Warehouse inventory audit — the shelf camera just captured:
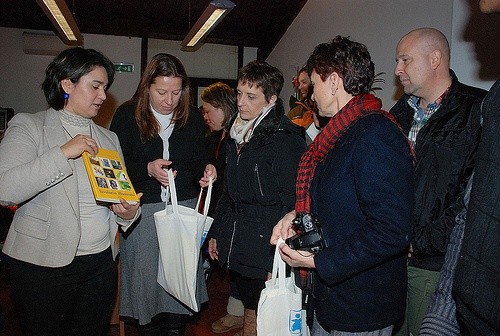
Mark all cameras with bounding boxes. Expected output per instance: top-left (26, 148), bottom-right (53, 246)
top-left (285, 212), bottom-right (328, 255)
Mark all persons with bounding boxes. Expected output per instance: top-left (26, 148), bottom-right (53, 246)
top-left (0, 0), bottom-right (500, 336)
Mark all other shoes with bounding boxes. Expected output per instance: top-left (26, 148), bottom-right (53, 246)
top-left (242, 314), bottom-right (257, 336)
top-left (212, 314), bottom-right (244, 333)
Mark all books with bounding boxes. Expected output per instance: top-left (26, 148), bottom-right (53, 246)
top-left (82, 148), bottom-right (140, 206)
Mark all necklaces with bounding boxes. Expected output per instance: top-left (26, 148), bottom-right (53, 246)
top-left (62, 122), bottom-right (93, 140)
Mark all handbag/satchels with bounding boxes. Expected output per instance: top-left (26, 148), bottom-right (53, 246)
top-left (257, 239), bottom-right (310, 336)
top-left (153, 169), bottom-right (214, 312)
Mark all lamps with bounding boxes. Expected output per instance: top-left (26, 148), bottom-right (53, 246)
top-left (180, 0), bottom-right (237, 53)
top-left (36, 0), bottom-right (84, 48)
